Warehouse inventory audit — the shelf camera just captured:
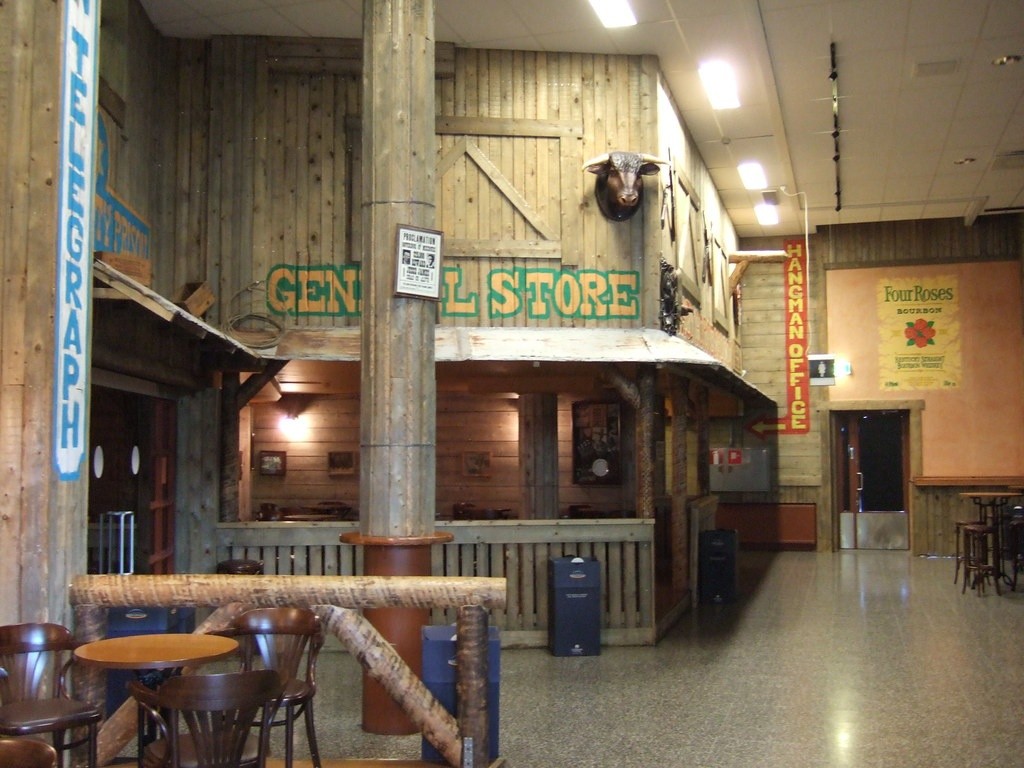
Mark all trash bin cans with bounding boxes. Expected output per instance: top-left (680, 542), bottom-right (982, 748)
top-left (420, 623), bottom-right (501, 762)
top-left (547, 555), bottom-right (602, 655)
top-left (106, 603), bottom-right (196, 721)
top-left (700, 528), bottom-right (739, 604)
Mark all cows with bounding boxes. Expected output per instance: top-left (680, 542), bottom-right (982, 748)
top-left (580, 150), bottom-right (672, 223)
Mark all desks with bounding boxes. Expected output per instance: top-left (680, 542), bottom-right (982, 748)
top-left (250, 500), bottom-right (638, 524)
top-left (959, 492), bottom-right (1024, 585)
top-left (72, 633), bottom-right (239, 768)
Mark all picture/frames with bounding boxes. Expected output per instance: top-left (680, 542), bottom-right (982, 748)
top-left (462, 450), bottom-right (492, 479)
top-left (259, 450), bottom-right (286, 476)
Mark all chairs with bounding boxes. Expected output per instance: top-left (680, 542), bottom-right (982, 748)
top-left (0, 607), bottom-right (323, 768)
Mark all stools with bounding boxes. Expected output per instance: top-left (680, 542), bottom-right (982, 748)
top-left (216, 560), bottom-right (263, 576)
top-left (954, 512), bottom-right (1024, 597)
